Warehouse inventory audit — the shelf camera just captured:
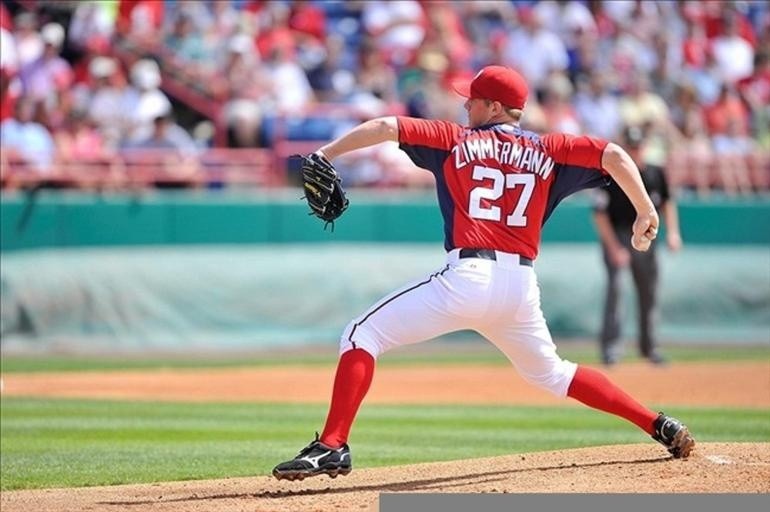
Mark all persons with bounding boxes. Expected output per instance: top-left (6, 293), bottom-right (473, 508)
top-left (269, 66), bottom-right (697, 483)
top-left (586, 123), bottom-right (685, 368)
top-left (1, 1), bottom-right (769, 200)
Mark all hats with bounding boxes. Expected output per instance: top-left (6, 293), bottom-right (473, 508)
top-left (621, 125), bottom-right (645, 147)
top-left (450, 65), bottom-right (527, 111)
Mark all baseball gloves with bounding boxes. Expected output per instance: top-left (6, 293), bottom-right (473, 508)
top-left (301, 151), bottom-right (350, 222)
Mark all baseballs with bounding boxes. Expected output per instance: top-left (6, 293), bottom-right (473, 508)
top-left (632, 235), bottom-right (651, 253)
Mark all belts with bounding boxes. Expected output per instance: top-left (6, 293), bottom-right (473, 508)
top-left (459, 247), bottom-right (532, 267)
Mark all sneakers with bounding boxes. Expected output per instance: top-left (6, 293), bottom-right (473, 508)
top-left (271, 431), bottom-right (353, 482)
top-left (600, 340), bottom-right (667, 367)
top-left (651, 409), bottom-right (695, 459)
